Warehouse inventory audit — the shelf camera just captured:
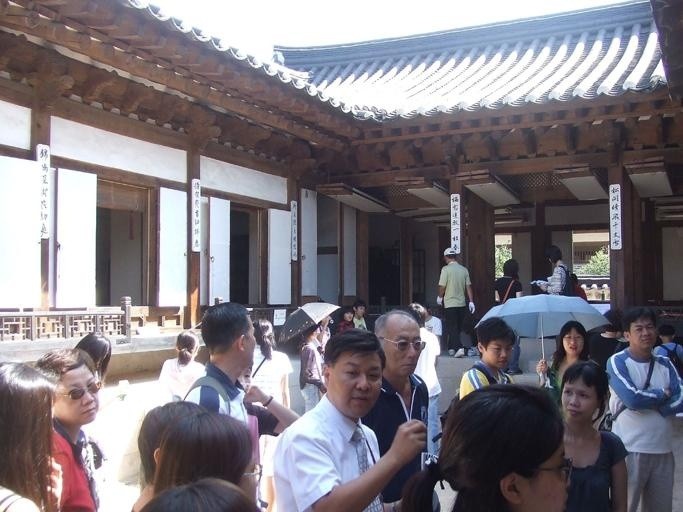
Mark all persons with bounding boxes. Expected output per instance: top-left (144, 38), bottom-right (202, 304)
top-left (435, 248), bottom-right (476, 358)
top-left (492, 259), bottom-right (524, 374)
top-left (1, 332), bottom-right (111, 511)
top-left (532, 245), bottom-right (569, 296)
top-left (132, 301), bottom-right (682, 511)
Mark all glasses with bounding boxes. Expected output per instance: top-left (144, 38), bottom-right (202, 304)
top-left (524, 456), bottom-right (572, 482)
top-left (62, 377), bottom-right (101, 399)
top-left (241, 463), bottom-right (262, 484)
top-left (378, 335), bottom-right (425, 351)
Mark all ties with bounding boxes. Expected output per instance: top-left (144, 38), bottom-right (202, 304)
top-left (352, 425), bottom-right (384, 512)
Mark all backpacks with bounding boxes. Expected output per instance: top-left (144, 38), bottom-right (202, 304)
top-left (658, 343), bottom-right (679, 372)
top-left (557, 265), bottom-right (587, 301)
top-left (432, 365), bottom-right (497, 443)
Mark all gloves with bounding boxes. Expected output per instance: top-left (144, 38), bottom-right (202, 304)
top-left (436, 295), bottom-right (442, 305)
top-left (468, 301), bottom-right (475, 314)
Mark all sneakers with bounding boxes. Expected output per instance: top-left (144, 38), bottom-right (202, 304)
top-left (453, 348), bottom-right (464, 357)
top-left (448, 348), bottom-right (455, 355)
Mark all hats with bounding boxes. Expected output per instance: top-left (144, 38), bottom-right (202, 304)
top-left (443, 247), bottom-right (455, 256)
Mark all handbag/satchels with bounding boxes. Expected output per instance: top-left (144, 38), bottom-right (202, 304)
top-left (596, 409), bottom-right (612, 432)
